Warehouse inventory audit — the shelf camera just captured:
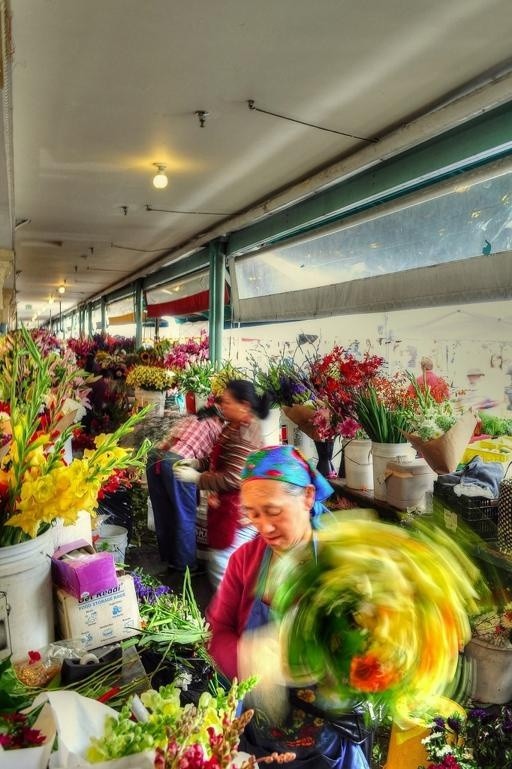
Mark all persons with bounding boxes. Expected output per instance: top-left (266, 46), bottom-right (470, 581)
top-left (203, 445), bottom-right (368, 769)
top-left (458, 367), bottom-right (485, 402)
top-left (171, 379), bottom-right (263, 593)
top-left (485, 353), bottom-right (506, 417)
top-left (146, 405), bottom-right (225, 578)
top-left (404, 356), bottom-right (450, 402)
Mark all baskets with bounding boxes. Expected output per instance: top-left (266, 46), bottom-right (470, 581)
top-left (497, 478), bottom-right (512, 555)
top-left (463, 434), bottom-right (511, 467)
top-left (432, 481), bottom-right (498, 546)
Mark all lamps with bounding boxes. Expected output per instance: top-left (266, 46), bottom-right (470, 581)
top-left (152, 163), bottom-right (168, 189)
top-left (27, 286), bottom-right (65, 326)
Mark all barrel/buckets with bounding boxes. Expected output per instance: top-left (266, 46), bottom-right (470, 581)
top-left (93, 523), bottom-right (128, 564)
top-left (341, 438), bottom-right (374, 490)
top-left (194, 489), bottom-right (216, 560)
top-left (258, 407), bottom-right (281, 446)
top-left (371, 441), bottom-right (417, 500)
top-left (194, 392), bottom-right (207, 413)
top-left (280, 408), bottom-right (314, 458)
top-left (464, 637), bottom-right (512, 705)
top-left (145, 496), bottom-right (156, 532)
top-left (134, 388), bottom-right (166, 420)
top-left (0, 524), bottom-right (54, 661)
